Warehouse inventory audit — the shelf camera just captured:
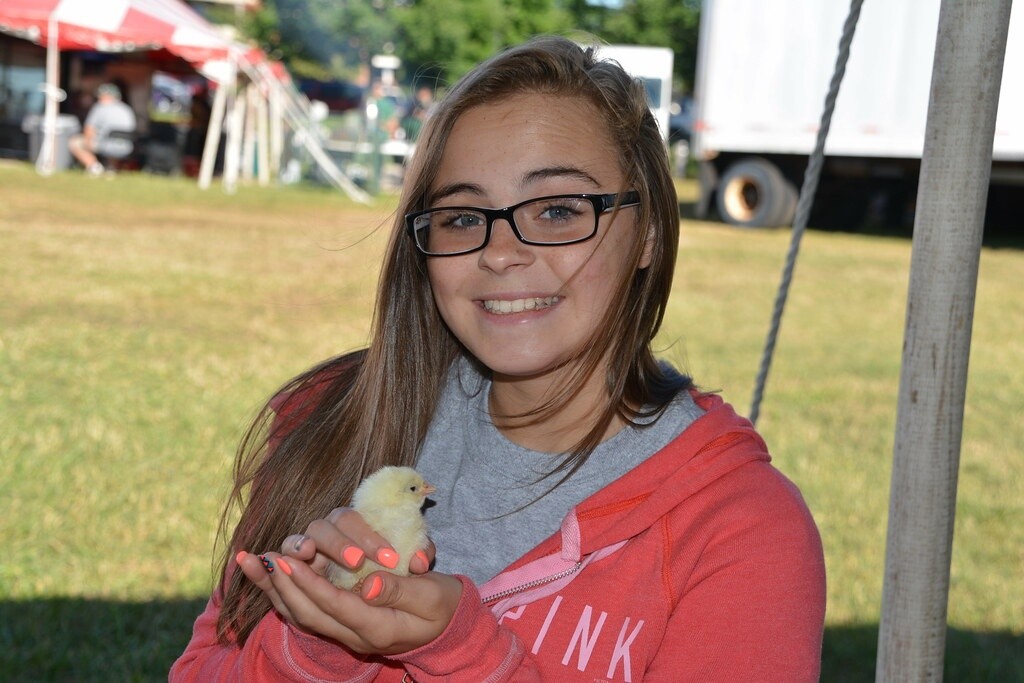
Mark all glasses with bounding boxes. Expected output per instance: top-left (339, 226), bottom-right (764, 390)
top-left (405, 190), bottom-right (641, 256)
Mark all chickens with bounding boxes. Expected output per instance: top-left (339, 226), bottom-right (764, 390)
top-left (303, 465), bottom-right (438, 594)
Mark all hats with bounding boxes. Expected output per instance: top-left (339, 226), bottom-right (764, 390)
top-left (96, 83), bottom-right (121, 97)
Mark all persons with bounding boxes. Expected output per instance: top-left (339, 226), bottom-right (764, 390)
top-left (168, 37), bottom-right (825, 683)
top-left (67, 84), bottom-right (138, 174)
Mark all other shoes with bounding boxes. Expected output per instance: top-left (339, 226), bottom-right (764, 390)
top-left (88, 163), bottom-right (105, 176)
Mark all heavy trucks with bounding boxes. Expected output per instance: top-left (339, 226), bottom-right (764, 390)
top-left (557, 0), bottom-right (1023, 227)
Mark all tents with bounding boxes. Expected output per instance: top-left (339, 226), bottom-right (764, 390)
top-left (0, 0), bottom-right (368, 200)
